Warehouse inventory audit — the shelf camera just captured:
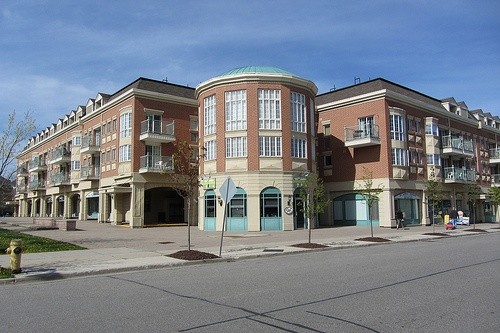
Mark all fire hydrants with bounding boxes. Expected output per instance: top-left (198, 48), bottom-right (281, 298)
top-left (4, 239), bottom-right (23, 274)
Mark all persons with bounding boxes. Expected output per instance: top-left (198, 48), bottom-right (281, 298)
top-left (449, 206), bottom-right (459, 228)
top-left (395, 208), bottom-right (405, 230)
